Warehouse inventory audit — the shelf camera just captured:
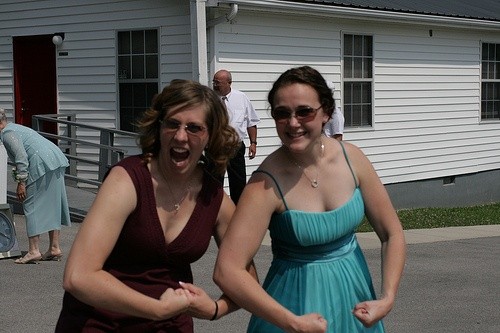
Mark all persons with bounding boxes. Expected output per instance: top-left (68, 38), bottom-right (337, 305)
top-left (55, 78), bottom-right (260, 333)
top-left (321, 80), bottom-right (345, 141)
top-left (206, 69), bottom-right (260, 207)
top-left (211, 65), bottom-right (407, 333)
top-left (0, 110), bottom-right (72, 265)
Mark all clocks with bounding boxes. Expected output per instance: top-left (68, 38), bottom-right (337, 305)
top-left (0, 204), bottom-right (22, 259)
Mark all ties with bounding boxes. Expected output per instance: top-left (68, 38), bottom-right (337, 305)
top-left (222, 97), bottom-right (227, 110)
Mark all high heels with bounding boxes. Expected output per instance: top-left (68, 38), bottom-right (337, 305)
top-left (14, 256), bottom-right (42, 264)
top-left (41, 253), bottom-right (62, 262)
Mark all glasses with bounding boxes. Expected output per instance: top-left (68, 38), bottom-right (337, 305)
top-left (271, 104), bottom-right (322, 119)
top-left (163, 119), bottom-right (206, 135)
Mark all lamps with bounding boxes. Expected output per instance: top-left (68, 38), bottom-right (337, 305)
top-left (52, 32), bottom-right (64, 45)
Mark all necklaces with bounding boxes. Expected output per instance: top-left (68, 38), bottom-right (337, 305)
top-left (282, 139), bottom-right (325, 187)
top-left (158, 159), bottom-right (194, 210)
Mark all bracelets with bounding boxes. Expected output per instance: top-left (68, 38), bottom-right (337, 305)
top-left (251, 141), bottom-right (256, 144)
top-left (210, 299), bottom-right (218, 321)
